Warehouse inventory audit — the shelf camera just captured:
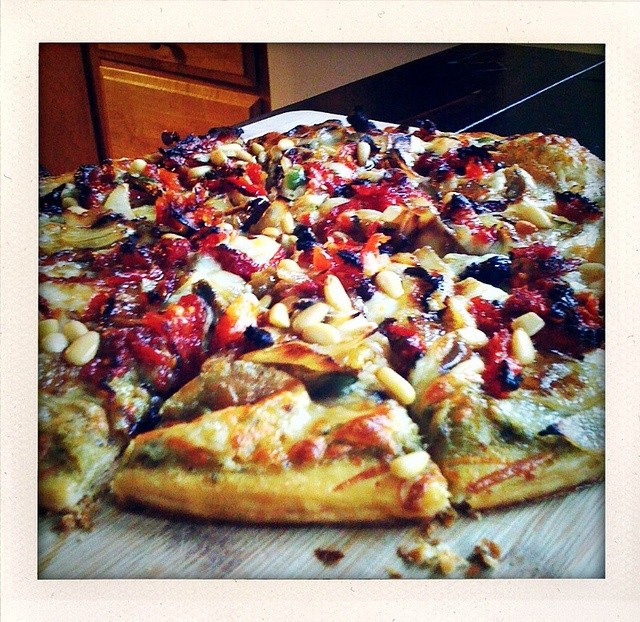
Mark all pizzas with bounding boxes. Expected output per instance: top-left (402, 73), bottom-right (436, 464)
top-left (38, 110), bottom-right (604, 527)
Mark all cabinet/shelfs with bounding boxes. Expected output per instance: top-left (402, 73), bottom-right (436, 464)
top-left (80, 38), bottom-right (264, 162)
